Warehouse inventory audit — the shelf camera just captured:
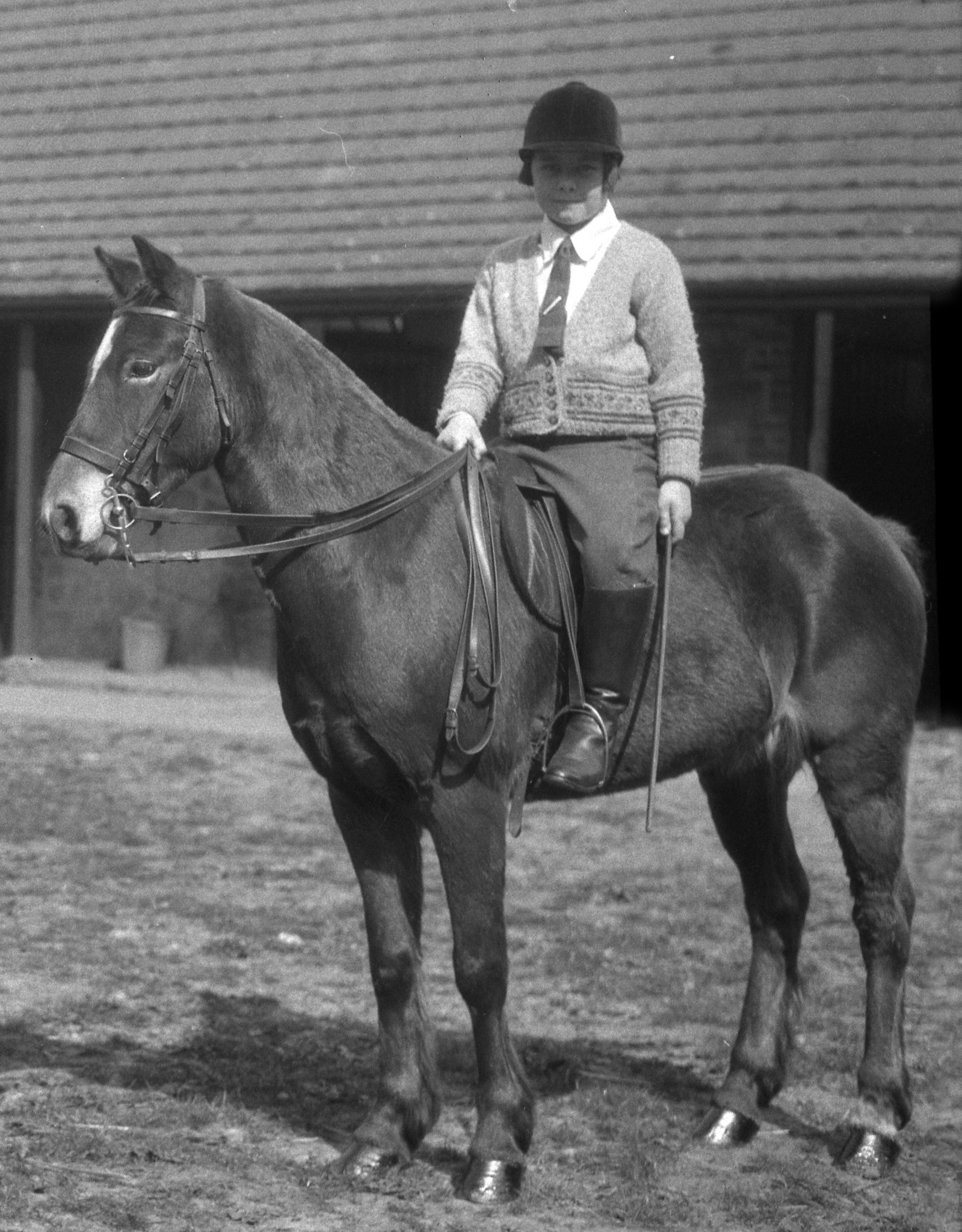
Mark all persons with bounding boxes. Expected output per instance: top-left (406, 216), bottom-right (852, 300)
top-left (433, 81), bottom-right (707, 793)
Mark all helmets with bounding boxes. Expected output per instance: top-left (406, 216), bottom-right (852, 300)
top-left (518, 82), bottom-right (623, 170)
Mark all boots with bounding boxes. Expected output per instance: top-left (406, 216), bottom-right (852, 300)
top-left (542, 585), bottom-right (654, 794)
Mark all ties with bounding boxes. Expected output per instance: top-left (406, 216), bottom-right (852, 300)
top-left (534, 236), bottom-right (571, 357)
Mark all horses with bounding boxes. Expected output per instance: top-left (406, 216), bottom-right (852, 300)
top-left (38, 234), bottom-right (928, 1208)
